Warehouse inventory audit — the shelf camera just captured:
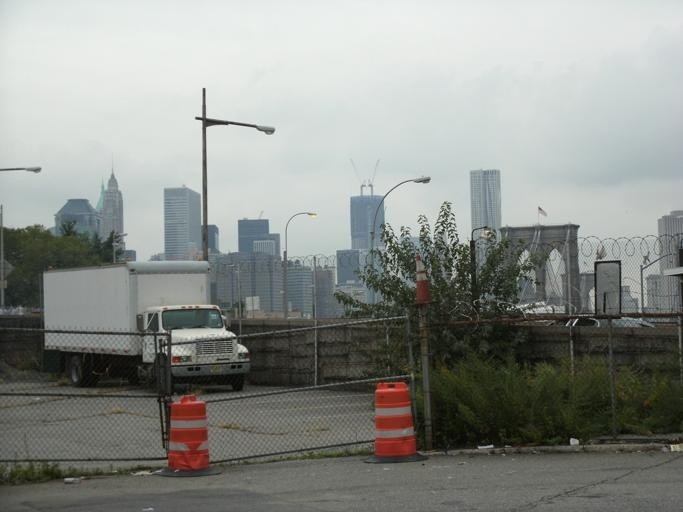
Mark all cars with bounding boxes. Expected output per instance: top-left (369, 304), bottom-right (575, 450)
top-left (545, 317), bottom-right (655, 328)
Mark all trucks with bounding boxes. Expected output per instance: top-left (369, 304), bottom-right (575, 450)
top-left (42, 261), bottom-right (250, 393)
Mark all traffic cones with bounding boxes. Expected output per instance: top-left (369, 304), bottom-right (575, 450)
top-left (410, 254), bottom-right (438, 307)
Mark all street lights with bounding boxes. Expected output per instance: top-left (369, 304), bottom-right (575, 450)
top-left (195, 115), bottom-right (275, 259)
top-left (1, 166), bottom-right (43, 174)
top-left (469, 226), bottom-right (494, 310)
top-left (282, 212), bottom-right (317, 318)
top-left (365, 177), bottom-right (431, 305)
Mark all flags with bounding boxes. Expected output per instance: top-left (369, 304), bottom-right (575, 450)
top-left (538, 207), bottom-right (548, 217)
